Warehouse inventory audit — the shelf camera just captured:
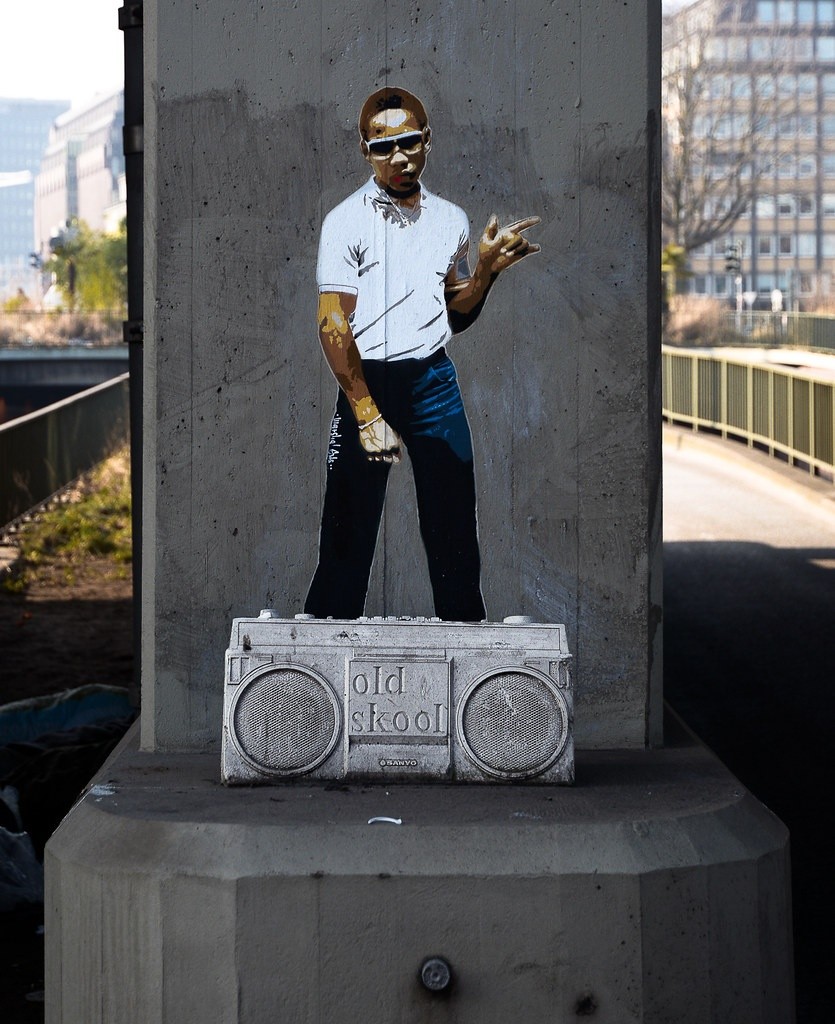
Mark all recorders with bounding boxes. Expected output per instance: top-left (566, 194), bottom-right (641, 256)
top-left (221, 607), bottom-right (575, 789)
